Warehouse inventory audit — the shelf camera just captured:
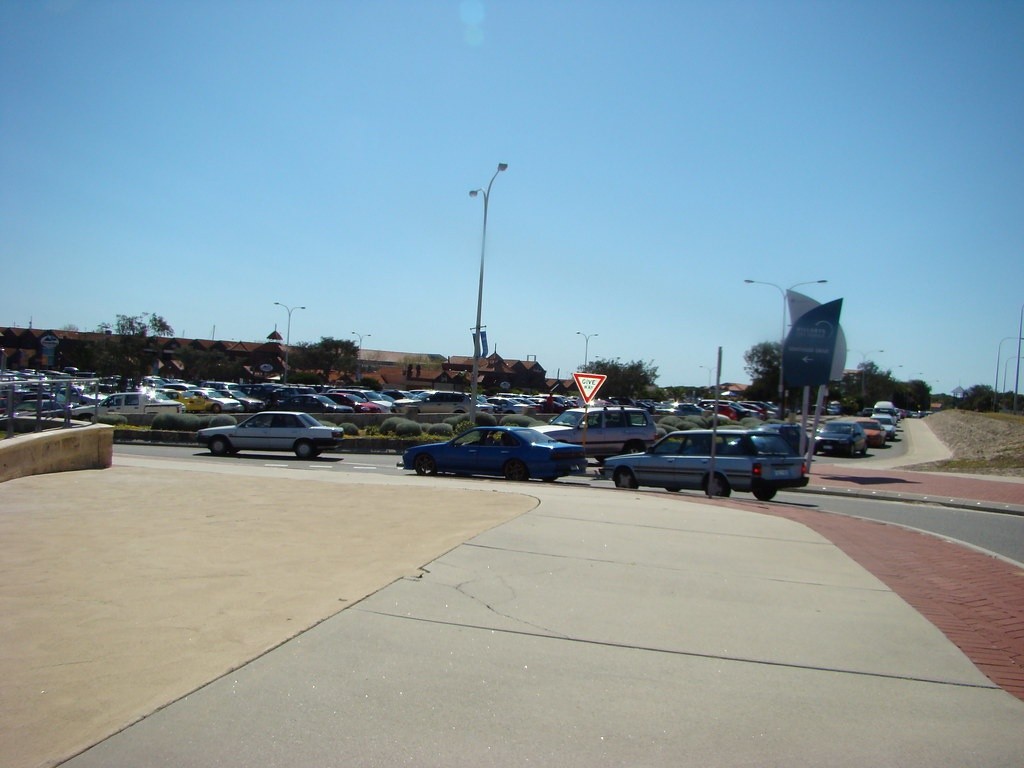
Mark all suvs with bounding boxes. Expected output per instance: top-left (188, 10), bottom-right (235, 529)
top-left (530, 406), bottom-right (657, 463)
top-left (390, 391), bottom-right (471, 414)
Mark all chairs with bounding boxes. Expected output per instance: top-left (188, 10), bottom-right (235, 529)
top-left (606, 414), bottom-right (614, 426)
top-left (500, 433), bottom-right (512, 446)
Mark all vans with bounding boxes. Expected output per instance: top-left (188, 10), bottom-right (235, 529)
top-left (873, 401), bottom-right (895, 417)
top-left (826, 400), bottom-right (841, 414)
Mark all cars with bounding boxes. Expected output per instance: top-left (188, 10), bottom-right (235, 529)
top-left (476, 392), bottom-right (790, 421)
top-left (155, 389), bottom-right (212, 413)
top-left (160, 378), bottom-right (415, 414)
top-left (812, 413), bottom-right (895, 457)
top-left (894, 407), bottom-right (926, 420)
top-left (863, 408), bottom-right (873, 416)
top-left (0, 367), bottom-right (161, 422)
top-left (196, 411), bottom-right (345, 461)
top-left (403, 425), bottom-right (587, 482)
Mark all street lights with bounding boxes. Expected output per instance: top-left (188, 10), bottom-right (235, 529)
top-left (274, 303), bottom-right (305, 385)
top-left (576, 332), bottom-right (598, 370)
top-left (469, 162), bottom-right (507, 424)
top-left (352, 332), bottom-right (371, 384)
top-left (745, 279), bottom-right (829, 420)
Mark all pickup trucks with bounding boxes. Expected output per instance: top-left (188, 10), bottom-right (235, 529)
top-left (71, 391), bottom-right (186, 417)
top-left (600, 428), bottom-right (809, 502)
